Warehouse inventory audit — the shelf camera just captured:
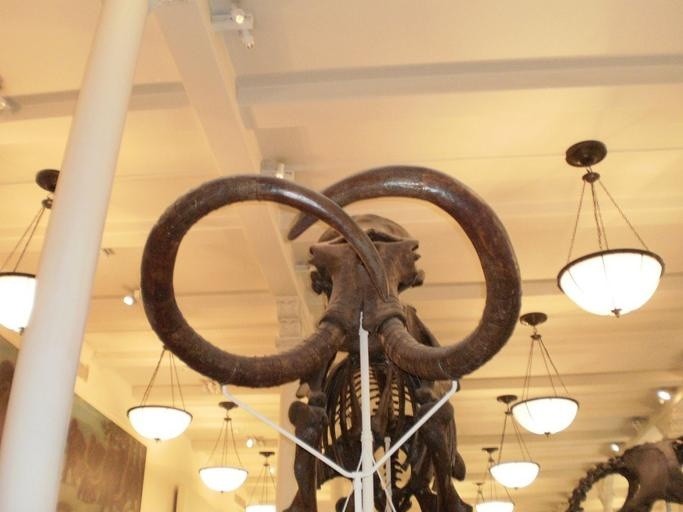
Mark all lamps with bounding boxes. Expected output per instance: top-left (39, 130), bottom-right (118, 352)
top-left (229, 4), bottom-right (256, 51)
top-left (472, 310), bottom-right (581, 511)
top-left (555, 139), bottom-right (666, 322)
top-left (125, 342), bottom-right (280, 512)
top-left (0, 169), bottom-right (59, 337)
